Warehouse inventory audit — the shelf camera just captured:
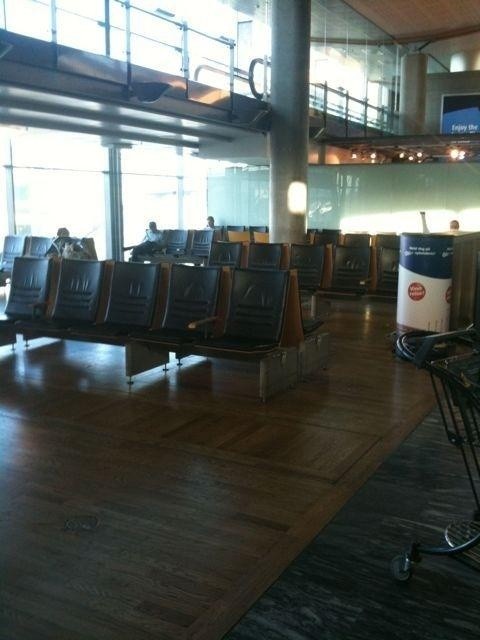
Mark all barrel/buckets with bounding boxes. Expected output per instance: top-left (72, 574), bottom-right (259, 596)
top-left (397, 232), bottom-right (453, 342)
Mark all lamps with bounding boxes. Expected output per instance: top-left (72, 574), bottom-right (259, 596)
top-left (122, 80), bottom-right (171, 103)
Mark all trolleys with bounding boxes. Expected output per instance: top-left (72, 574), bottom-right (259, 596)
top-left (389, 321), bottom-right (480, 581)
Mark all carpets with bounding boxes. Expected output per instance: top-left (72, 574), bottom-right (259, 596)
top-left (216, 384), bottom-right (480, 640)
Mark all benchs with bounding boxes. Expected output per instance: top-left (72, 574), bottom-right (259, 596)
top-left (1, 255), bottom-right (333, 398)
top-left (1, 226), bottom-right (398, 305)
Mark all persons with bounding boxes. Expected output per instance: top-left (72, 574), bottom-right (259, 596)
top-left (449, 220), bottom-right (460, 235)
top-left (131, 222), bottom-right (168, 259)
top-left (201, 217), bottom-right (220, 231)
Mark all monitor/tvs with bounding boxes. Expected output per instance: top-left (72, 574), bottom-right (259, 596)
top-left (440, 92), bottom-right (480, 134)
